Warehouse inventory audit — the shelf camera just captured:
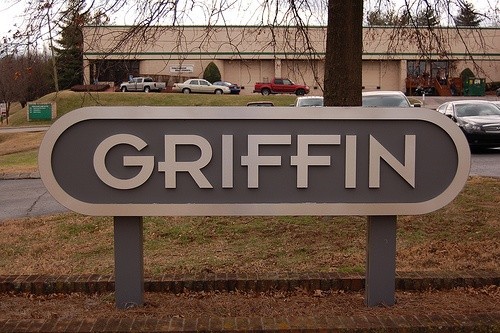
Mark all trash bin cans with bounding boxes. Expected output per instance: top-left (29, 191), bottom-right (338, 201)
top-left (462, 77), bottom-right (486, 96)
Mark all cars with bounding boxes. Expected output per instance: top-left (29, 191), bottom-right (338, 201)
top-left (171, 78), bottom-right (231, 95)
top-left (434, 99), bottom-right (500, 153)
top-left (211, 80), bottom-right (241, 95)
top-left (289, 95), bottom-right (323, 107)
top-left (245, 101), bottom-right (274, 107)
top-left (362, 90), bottom-right (422, 109)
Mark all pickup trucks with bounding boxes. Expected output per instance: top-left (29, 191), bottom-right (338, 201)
top-left (119, 76), bottom-right (167, 93)
top-left (252, 77), bottom-right (310, 96)
